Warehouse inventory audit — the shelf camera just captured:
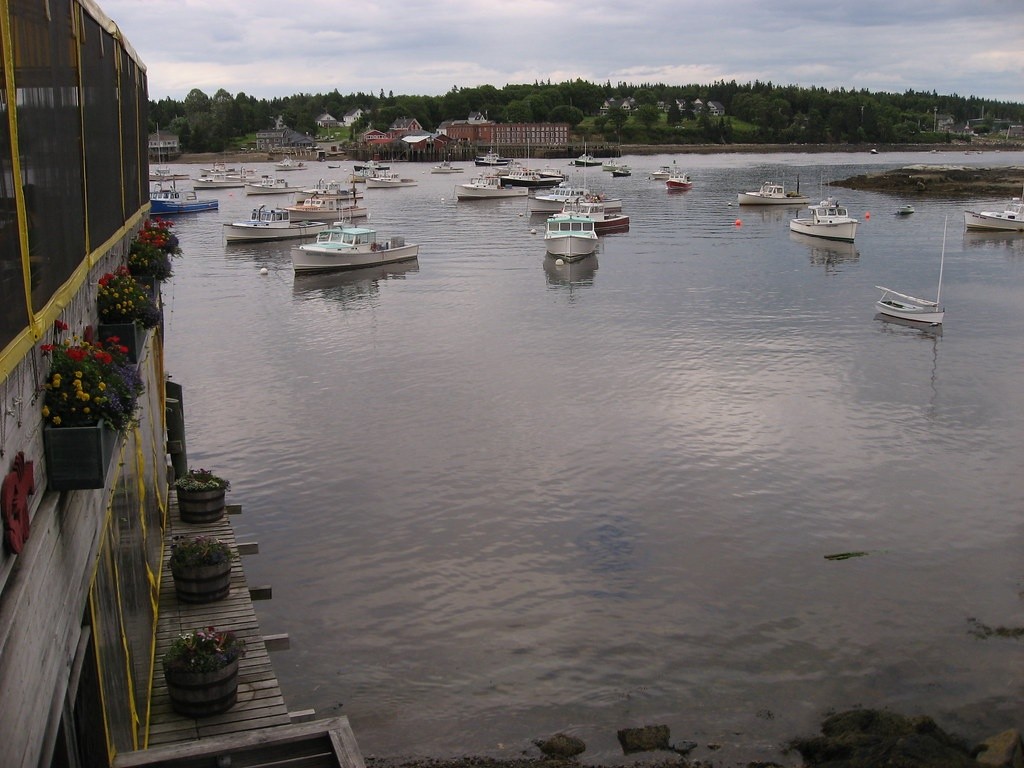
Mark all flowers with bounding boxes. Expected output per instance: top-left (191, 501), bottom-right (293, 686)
top-left (38, 317), bottom-right (147, 442)
top-left (174, 468), bottom-right (232, 493)
top-left (169, 534), bottom-right (236, 568)
top-left (161, 624), bottom-right (248, 677)
top-left (96, 213), bottom-right (183, 332)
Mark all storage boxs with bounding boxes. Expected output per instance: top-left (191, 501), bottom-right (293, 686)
top-left (129, 272), bottom-right (160, 302)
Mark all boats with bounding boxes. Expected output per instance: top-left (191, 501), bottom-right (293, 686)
top-left (455, 177), bottom-right (529, 200)
top-left (553, 202), bottom-right (629, 230)
top-left (529, 188), bottom-right (622, 213)
top-left (897, 204), bottom-right (916, 215)
top-left (737, 183), bottom-right (807, 205)
top-left (543, 214), bottom-right (598, 257)
top-left (603, 159), bottom-right (622, 171)
top-left (149, 154), bottom-right (564, 224)
top-left (224, 203), bottom-right (330, 243)
top-left (789, 199), bottom-right (859, 243)
top-left (613, 169), bottom-right (631, 177)
top-left (575, 154), bottom-right (602, 166)
top-left (666, 171), bottom-right (691, 190)
top-left (290, 226), bottom-right (419, 273)
top-left (874, 284), bottom-right (947, 326)
top-left (652, 160), bottom-right (691, 179)
top-left (961, 200), bottom-right (1024, 232)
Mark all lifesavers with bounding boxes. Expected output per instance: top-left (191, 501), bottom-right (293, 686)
top-left (175, 193), bottom-right (179, 198)
top-left (532, 176), bottom-right (535, 180)
top-left (160, 190), bottom-right (171, 192)
top-left (586, 194), bottom-right (590, 199)
top-left (371, 242), bottom-right (377, 251)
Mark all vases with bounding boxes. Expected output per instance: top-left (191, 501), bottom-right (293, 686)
top-left (42, 415), bottom-right (114, 489)
top-left (164, 655), bottom-right (240, 717)
top-left (172, 559), bottom-right (230, 603)
top-left (176, 486), bottom-right (226, 522)
top-left (98, 318), bottom-right (146, 363)
